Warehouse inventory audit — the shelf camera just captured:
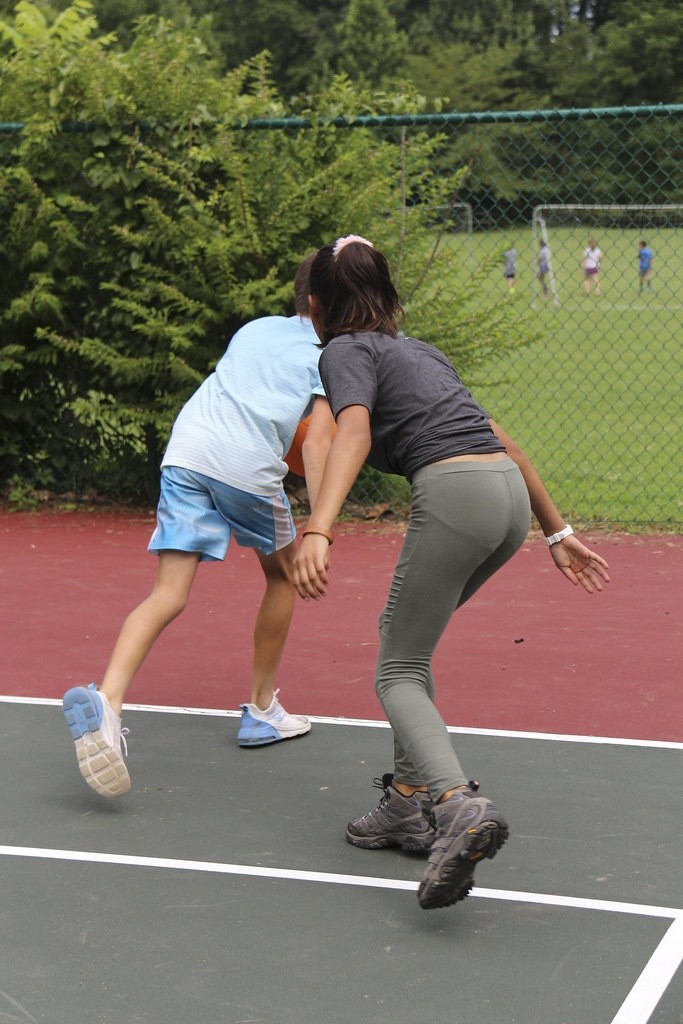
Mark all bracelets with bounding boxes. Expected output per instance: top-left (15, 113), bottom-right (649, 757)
top-left (301, 526), bottom-right (334, 546)
top-left (544, 523), bottom-right (575, 546)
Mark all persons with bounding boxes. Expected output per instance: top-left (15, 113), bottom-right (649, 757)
top-left (61, 255), bottom-right (334, 796)
top-left (501, 242), bottom-right (518, 292)
top-left (581, 240), bottom-right (604, 297)
top-left (535, 240), bottom-right (553, 297)
top-left (637, 239), bottom-right (654, 295)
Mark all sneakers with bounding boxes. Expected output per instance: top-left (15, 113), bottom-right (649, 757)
top-left (237, 693), bottom-right (311, 747)
top-left (346, 773), bottom-right (438, 852)
top-left (417, 786), bottom-right (509, 910)
top-left (61, 686), bottom-right (131, 795)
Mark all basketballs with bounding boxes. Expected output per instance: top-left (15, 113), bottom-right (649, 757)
top-left (281, 411), bottom-right (339, 479)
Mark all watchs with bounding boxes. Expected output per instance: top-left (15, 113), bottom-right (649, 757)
top-left (288, 234), bottom-right (613, 911)
